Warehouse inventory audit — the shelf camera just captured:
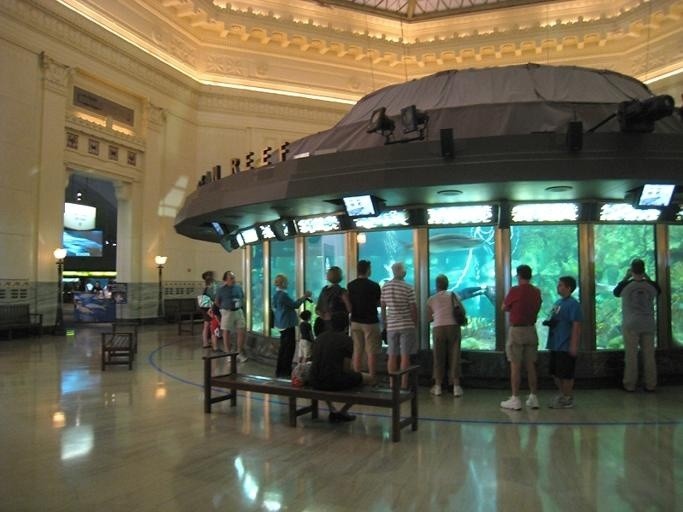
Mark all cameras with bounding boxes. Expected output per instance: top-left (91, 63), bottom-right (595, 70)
top-left (543, 316), bottom-right (559, 328)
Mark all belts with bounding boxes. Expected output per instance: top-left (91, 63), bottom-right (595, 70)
top-left (223, 308), bottom-right (242, 312)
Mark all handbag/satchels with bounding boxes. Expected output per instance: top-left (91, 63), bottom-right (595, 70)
top-left (197, 295), bottom-right (216, 309)
top-left (314, 317), bottom-right (324, 336)
top-left (453, 306), bottom-right (468, 326)
top-left (328, 296), bottom-right (346, 312)
top-left (291, 361), bottom-right (313, 388)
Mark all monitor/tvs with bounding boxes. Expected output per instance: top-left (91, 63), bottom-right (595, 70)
top-left (60, 231), bottom-right (104, 257)
top-left (638, 184), bottom-right (676, 210)
top-left (211, 222), bottom-right (227, 236)
top-left (342, 195), bottom-right (374, 218)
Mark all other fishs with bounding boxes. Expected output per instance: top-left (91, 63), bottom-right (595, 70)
top-left (393, 234), bottom-right (538, 306)
top-left (481, 326), bottom-right (495, 337)
top-left (538, 267), bottom-right (561, 276)
top-left (596, 282), bottom-right (617, 294)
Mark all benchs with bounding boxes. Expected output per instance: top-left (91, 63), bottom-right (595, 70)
top-left (0, 302), bottom-right (43, 340)
top-left (163, 298), bottom-right (202, 323)
top-left (100, 323), bottom-right (138, 370)
top-left (201, 351), bottom-right (420, 443)
top-left (176, 311), bottom-right (203, 336)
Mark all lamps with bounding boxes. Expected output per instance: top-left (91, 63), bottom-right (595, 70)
top-left (366, 103), bottom-right (430, 145)
top-left (618, 90), bottom-right (676, 135)
top-left (563, 117), bottom-right (584, 151)
top-left (153, 255), bottom-right (168, 325)
top-left (51, 247), bottom-right (67, 336)
top-left (438, 125), bottom-right (454, 159)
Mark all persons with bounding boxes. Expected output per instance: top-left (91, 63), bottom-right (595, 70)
top-left (613, 258), bottom-right (662, 391)
top-left (543, 277), bottom-right (586, 408)
top-left (271, 260), bottom-right (417, 423)
top-left (500, 265), bottom-right (542, 409)
top-left (425, 273), bottom-right (465, 397)
top-left (92, 281), bottom-right (104, 296)
top-left (197, 270), bottom-right (249, 363)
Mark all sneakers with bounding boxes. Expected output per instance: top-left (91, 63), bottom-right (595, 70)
top-left (237, 354), bottom-right (250, 363)
top-left (500, 400), bottom-right (521, 411)
top-left (400, 387), bottom-right (411, 394)
top-left (526, 398), bottom-right (539, 409)
top-left (454, 385), bottom-right (464, 396)
top-left (329, 411), bottom-right (356, 422)
top-left (550, 393), bottom-right (576, 408)
top-left (430, 384), bottom-right (442, 396)
